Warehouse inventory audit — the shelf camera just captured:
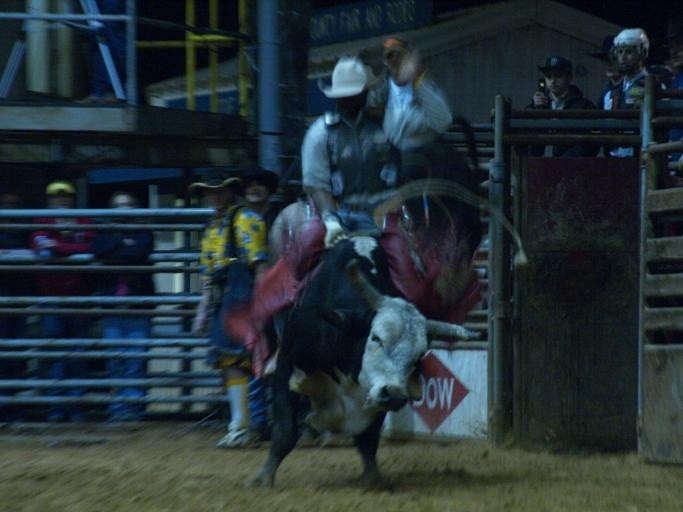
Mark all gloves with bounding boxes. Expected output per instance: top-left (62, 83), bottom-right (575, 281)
top-left (323, 215), bottom-right (348, 248)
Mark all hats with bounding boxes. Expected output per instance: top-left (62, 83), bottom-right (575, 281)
top-left (239, 165), bottom-right (279, 192)
top-left (381, 37), bottom-right (411, 59)
top-left (185, 166), bottom-right (241, 201)
top-left (536, 56), bottom-right (573, 76)
top-left (315, 56), bottom-right (369, 102)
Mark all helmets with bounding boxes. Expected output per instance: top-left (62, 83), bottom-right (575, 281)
top-left (611, 26), bottom-right (651, 58)
top-left (44, 179), bottom-right (77, 198)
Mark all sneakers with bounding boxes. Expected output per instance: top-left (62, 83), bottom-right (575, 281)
top-left (215, 424), bottom-right (253, 450)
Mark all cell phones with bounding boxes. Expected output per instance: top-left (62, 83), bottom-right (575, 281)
top-left (539, 77), bottom-right (545, 94)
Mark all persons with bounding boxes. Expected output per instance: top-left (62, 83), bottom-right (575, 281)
top-left (0, 190), bottom-right (38, 422)
top-left (88, 192), bottom-right (156, 422)
top-left (27, 181), bottom-right (98, 423)
top-left (289, 57), bottom-right (455, 388)
top-left (233, 167), bottom-right (309, 449)
top-left (368, 36), bottom-right (437, 212)
top-left (513, 26), bottom-right (683, 177)
top-left (186, 164), bottom-right (272, 448)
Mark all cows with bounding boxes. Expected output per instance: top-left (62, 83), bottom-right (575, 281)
top-left (243, 234), bottom-right (484, 490)
